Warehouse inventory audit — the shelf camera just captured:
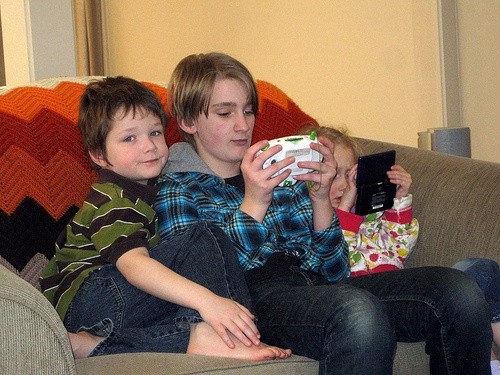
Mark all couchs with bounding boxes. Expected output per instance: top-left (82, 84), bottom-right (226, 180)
top-left (0, 77), bottom-right (500, 375)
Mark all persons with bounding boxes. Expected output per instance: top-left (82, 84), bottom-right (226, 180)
top-left (36, 74), bottom-right (292, 361)
top-left (296, 125), bottom-right (500, 375)
top-left (155, 51), bottom-right (493, 375)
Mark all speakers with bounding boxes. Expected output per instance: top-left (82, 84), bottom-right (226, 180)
top-left (417, 126), bottom-right (471, 158)
